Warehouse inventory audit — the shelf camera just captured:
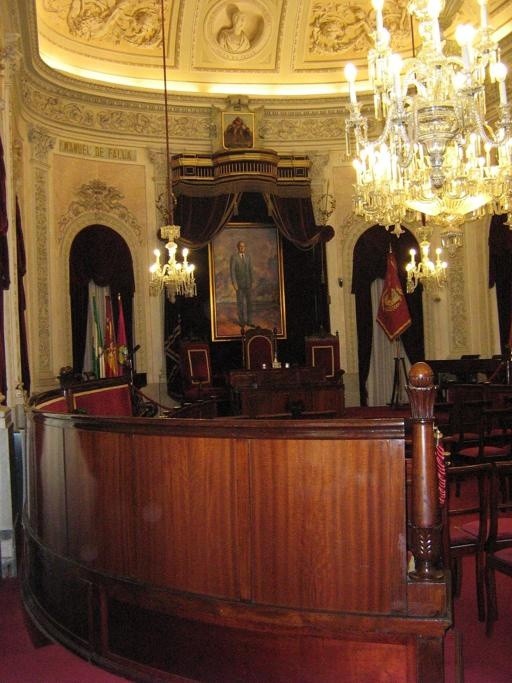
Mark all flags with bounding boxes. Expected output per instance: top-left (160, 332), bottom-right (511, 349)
top-left (91, 292), bottom-right (103, 376)
top-left (102, 296), bottom-right (119, 376)
top-left (115, 298), bottom-right (128, 375)
top-left (373, 250), bottom-right (411, 345)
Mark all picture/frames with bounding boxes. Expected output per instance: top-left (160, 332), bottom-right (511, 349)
top-left (207, 223), bottom-right (287, 343)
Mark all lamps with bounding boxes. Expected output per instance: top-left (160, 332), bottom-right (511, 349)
top-left (144, 2), bottom-right (199, 300)
top-left (342, 1), bottom-right (512, 298)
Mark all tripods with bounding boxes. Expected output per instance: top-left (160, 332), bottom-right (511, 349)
top-left (391, 336), bottom-right (409, 407)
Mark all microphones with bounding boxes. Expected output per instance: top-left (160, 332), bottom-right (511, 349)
top-left (127, 345), bottom-right (141, 359)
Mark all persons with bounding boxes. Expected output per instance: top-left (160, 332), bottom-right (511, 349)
top-left (230, 238), bottom-right (255, 326)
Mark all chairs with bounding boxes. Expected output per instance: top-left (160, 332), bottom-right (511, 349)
top-left (178, 324), bottom-right (346, 389)
top-left (424, 399), bottom-right (512, 638)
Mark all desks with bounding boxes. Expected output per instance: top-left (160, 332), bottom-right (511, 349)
top-left (444, 382), bottom-right (511, 403)
top-left (224, 367), bottom-right (327, 411)
